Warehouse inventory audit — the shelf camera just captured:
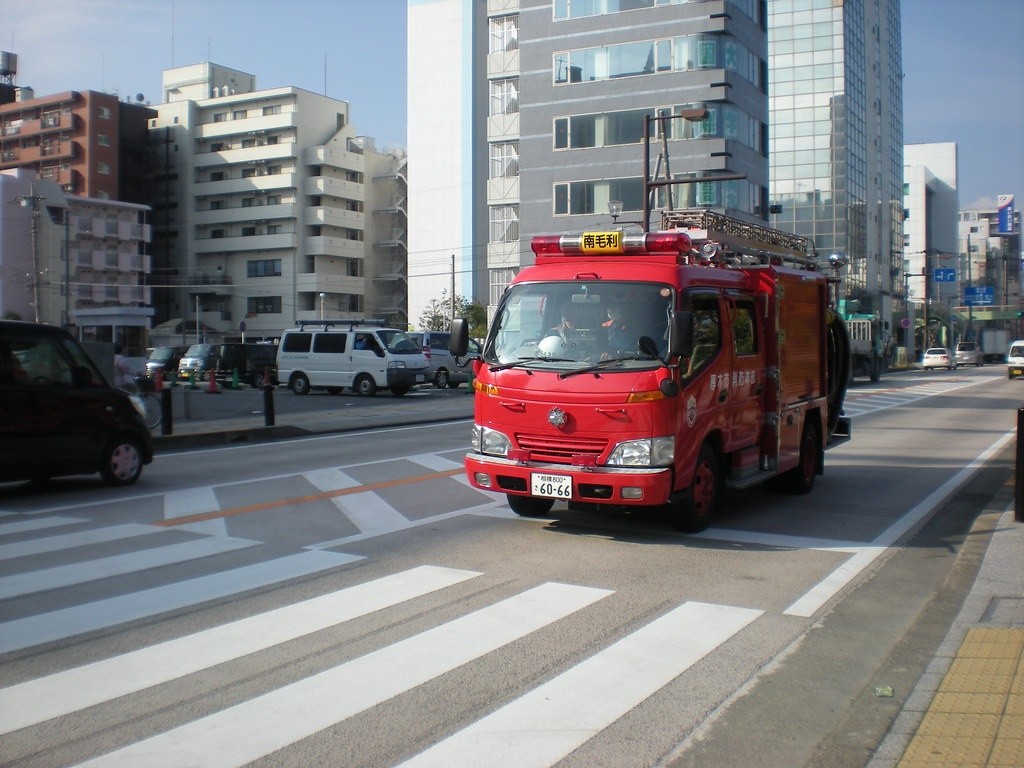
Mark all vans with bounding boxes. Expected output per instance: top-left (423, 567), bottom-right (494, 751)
top-left (275, 319), bottom-right (428, 398)
top-left (145, 345), bottom-right (192, 380)
top-left (1007, 340), bottom-right (1024, 380)
top-left (180, 342), bottom-right (223, 381)
top-left (390, 331), bottom-right (483, 390)
top-left (955, 341), bottom-right (984, 367)
top-left (0, 318), bottom-right (155, 487)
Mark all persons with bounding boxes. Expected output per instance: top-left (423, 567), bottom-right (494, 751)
top-left (114, 343), bottom-right (142, 394)
top-left (543, 302), bottom-right (641, 360)
top-left (355, 334), bottom-right (367, 350)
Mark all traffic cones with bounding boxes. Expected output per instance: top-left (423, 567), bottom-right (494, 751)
top-left (205, 368), bottom-right (227, 396)
top-left (263, 366), bottom-right (274, 388)
top-left (229, 367), bottom-right (242, 391)
top-left (154, 367), bottom-right (165, 393)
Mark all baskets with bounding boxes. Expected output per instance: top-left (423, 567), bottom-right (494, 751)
top-left (134, 376), bottom-right (155, 392)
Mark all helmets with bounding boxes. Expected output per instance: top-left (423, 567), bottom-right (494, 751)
top-left (538, 336), bottom-right (567, 357)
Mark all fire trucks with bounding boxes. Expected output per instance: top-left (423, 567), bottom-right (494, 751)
top-left (469, 208), bottom-right (853, 534)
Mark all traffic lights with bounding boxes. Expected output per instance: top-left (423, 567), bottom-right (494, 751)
top-left (1017, 312), bottom-right (1024, 316)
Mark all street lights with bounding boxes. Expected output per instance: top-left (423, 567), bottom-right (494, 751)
top-left (320, 292), bottom-right (327, 328)
top-left (20, 183), bottom-right (44, 324)
top-left (642, 109), bottom-right (712, 237)
top-left (196, 295), bottom-right (202, 344)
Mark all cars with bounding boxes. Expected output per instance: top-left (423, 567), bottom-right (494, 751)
top-left (925, 348), bottom-right (958, 369)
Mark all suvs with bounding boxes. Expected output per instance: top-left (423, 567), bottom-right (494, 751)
top-left (219, 342), bottom-right (280, 390)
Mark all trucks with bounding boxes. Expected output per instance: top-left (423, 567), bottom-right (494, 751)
top-left (982, 328), bottom-right (1012, 364)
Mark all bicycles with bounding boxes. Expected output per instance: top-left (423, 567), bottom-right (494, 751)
top-left (122, 376), bottom-right (163, 429)
